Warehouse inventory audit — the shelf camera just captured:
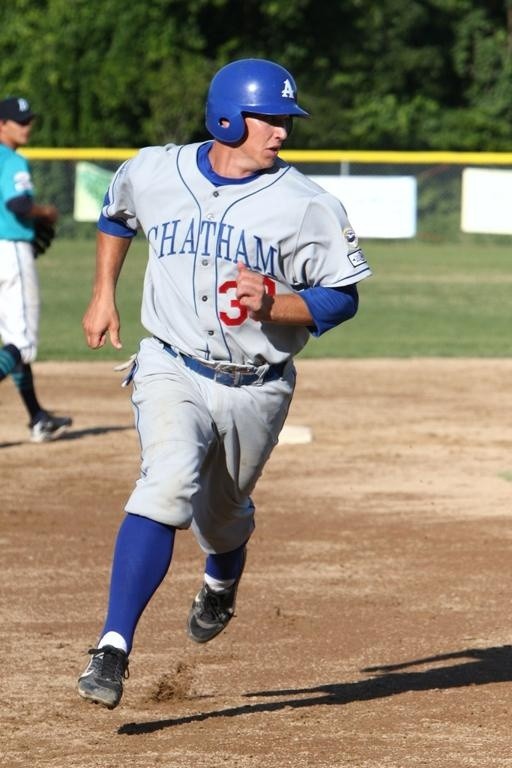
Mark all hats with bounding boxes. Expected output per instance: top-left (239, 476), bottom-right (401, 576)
top-left (0, 97), bottom-right (42, 124)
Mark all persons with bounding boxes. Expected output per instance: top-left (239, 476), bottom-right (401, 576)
top-left (77, 57), bottom-right (373, 711)
top-left (2, 96), bottom-right (75, 443)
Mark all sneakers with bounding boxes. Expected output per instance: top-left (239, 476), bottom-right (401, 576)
top-left (26, 410), bottom-right (73, 445)
top-left (186, 545), bottom-right (250, 644)
top-left (76, 645), bottom-right (131, 709)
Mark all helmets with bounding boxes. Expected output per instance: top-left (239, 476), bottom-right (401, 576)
top-left (202, 57), bottom-right (315, 147)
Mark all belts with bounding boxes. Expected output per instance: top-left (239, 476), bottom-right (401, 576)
top-left (149, 333), bottom-right (289, 390)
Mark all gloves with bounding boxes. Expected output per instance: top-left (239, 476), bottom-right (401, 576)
top-left (114, 353), bottom-right (140, 391)
